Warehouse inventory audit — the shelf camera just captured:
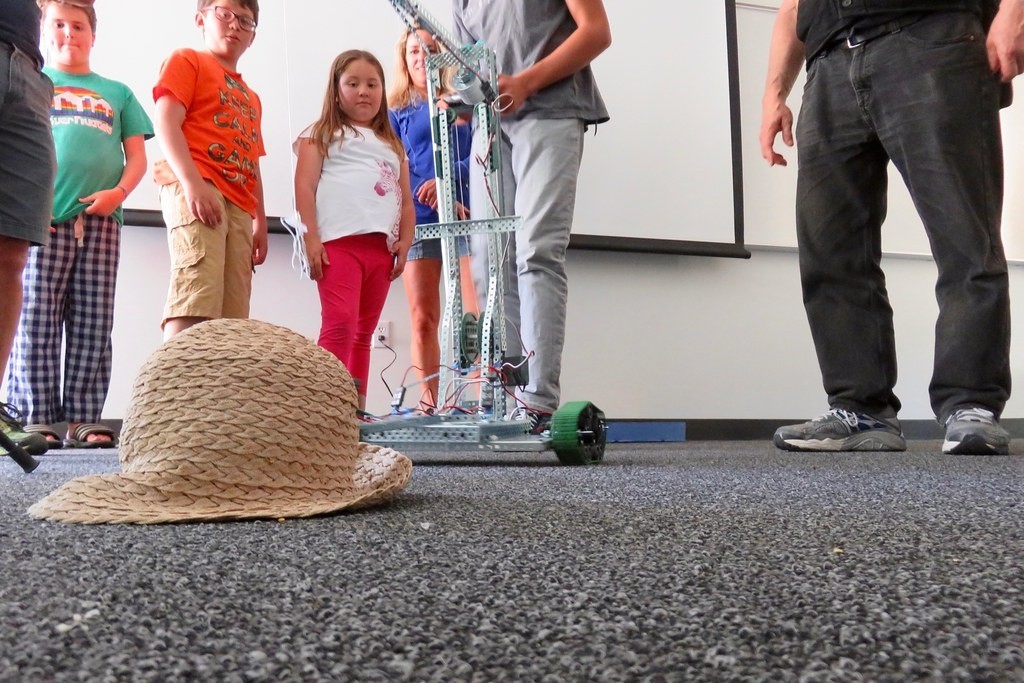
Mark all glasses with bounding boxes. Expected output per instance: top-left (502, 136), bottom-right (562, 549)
top-left (203, 5), bottom-right (258, 32)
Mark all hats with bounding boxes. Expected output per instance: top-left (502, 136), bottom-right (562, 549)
top-left (28, 316), bottom-right (414, 525)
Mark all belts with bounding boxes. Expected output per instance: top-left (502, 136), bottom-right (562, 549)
top-left (828, 13), bottom-right (932, 49)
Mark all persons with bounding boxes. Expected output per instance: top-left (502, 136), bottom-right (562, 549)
top-left (0, 0), bottom-right (156, 456)
top-left (292, 0), bottom-right (611, 435)
top-left (761, 0), bottom-right (1024, 456)
top-left (152, 0), bottom-right (268, 345)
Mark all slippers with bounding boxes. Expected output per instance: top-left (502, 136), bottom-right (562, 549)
top-left (23, 422), bottom-right (62, 448)
top-left (63, 420), bottom-right (118, 449)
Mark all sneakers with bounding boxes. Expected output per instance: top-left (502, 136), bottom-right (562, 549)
top-left (0, 401), bottom-right (49, 456)
top-left (772, 405), bottom-right (908, 453)
top-left (942, 407), bottom-right (1011, 456)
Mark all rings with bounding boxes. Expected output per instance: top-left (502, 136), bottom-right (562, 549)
top-left (425, 198), bottom-right (429, 202)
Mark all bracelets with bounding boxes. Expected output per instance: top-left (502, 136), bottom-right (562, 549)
top-left (117, 186), bottom-right (128, 198)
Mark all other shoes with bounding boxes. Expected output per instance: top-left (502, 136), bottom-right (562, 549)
top-left (511, 408), bottom-right (552, 435)
